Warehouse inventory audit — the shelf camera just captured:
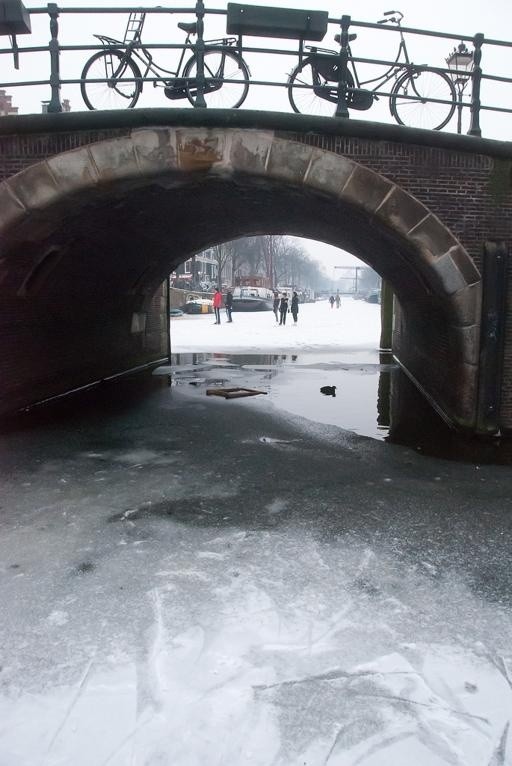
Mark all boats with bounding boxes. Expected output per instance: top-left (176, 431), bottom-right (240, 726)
top-left (349, 285), bottom-right (383, 305)
top-left (169, 273), bottom-right (329, 317)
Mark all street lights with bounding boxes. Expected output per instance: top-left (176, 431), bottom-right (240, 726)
top-left (445, 41), bottom-right (477, 136)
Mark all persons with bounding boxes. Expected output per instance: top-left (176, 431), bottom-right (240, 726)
top-left (329, 294), bottom-right (341, 309)
top-left (273, 291), bottom-right (298, 326)
top-left (212, 288), bottom-right (233, 324)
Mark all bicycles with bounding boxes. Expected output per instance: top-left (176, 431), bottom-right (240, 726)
top-left (281, 5), bottom-right (460, 132)
top-left (78, 8), bottom-right (256, 109)
